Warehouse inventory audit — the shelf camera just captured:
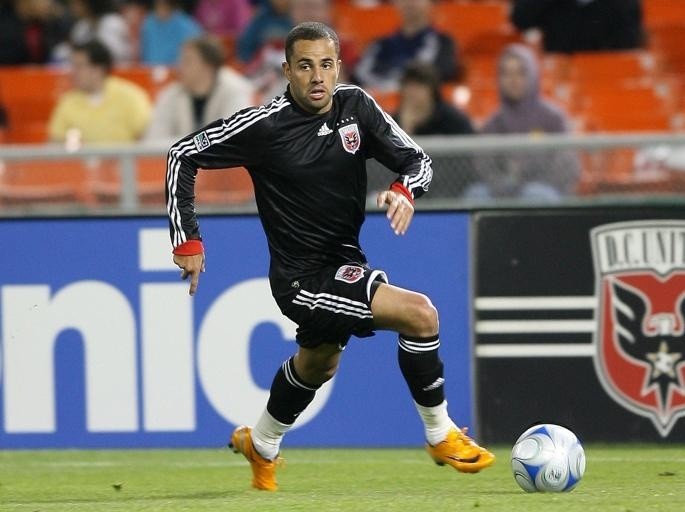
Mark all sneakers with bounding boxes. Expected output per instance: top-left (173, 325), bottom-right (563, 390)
top-left (425, 425), bottom-right (496, 474)
top-left (228, 426), bottom-right (284, 491)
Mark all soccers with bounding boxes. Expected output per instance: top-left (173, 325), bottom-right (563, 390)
top-left (511, 423), bottom-right (586, 492)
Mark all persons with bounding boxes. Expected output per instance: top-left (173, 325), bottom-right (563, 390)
top-left (166, 22), bottom-right (496, 491)
